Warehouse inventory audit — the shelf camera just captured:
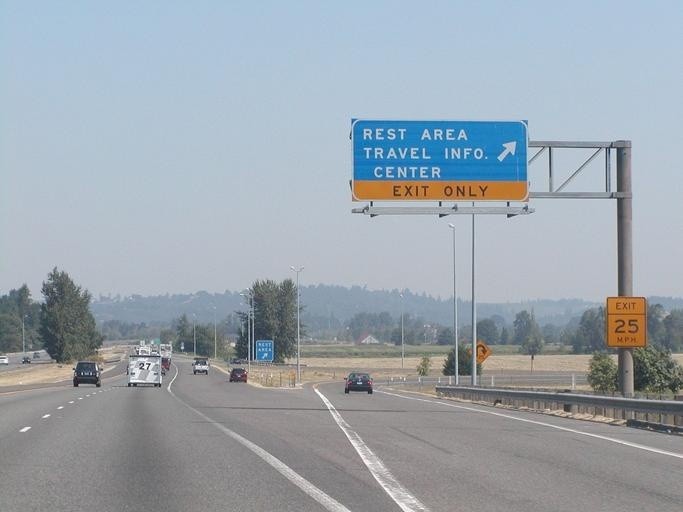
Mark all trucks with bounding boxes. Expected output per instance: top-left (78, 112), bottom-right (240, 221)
top-left (127, 343), bottom-right (172, 387)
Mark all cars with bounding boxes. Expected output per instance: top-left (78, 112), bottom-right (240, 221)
top-left (192, 357), bottom-right (210, 375)
top-left (230, 367), bottom-right (247, 382)
top-left (73, 361), bottom-right (103, 387)
top-left (345, 371), bottom-right (373, 394)
top-left (21, 355), bottom-right (31, 364)
top-left (0, 356), bottom-right (8, 365)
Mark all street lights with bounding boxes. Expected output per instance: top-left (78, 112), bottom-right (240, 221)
top-left (400, 294), bottom-right (404, 369)
top-left (448, 223), bottom-right (458, 385)
top-left (240, 288), bottom-right (255, 361)
top-left (290, 265), bottom-right (305, 383)
